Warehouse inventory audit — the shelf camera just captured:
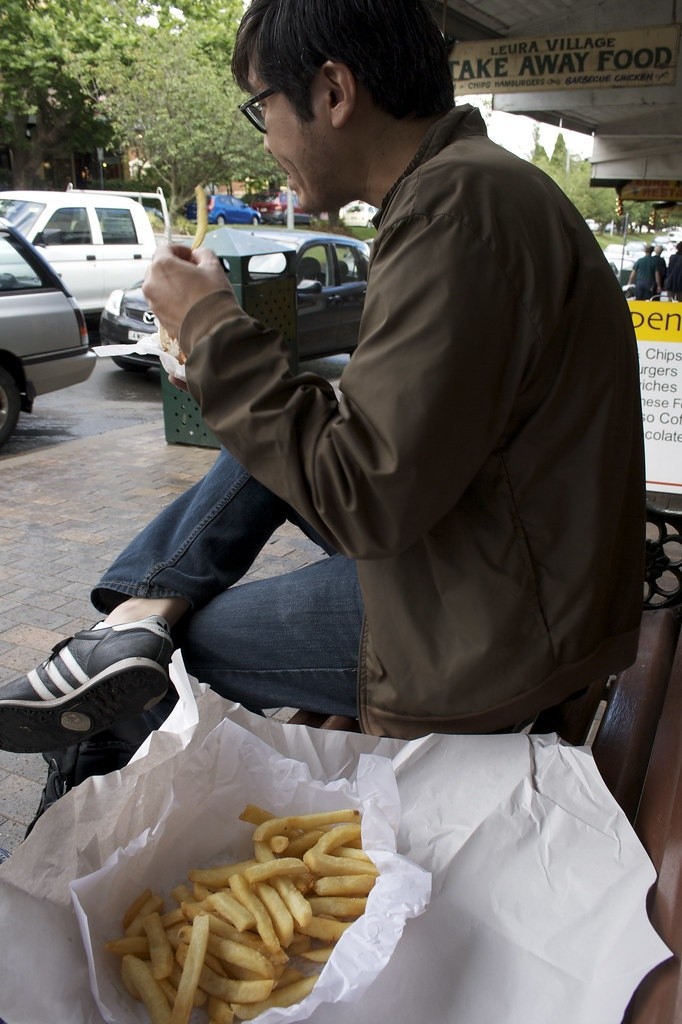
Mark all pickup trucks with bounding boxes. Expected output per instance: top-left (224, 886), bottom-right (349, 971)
top-left (0, 182), bottom-right (199, 317)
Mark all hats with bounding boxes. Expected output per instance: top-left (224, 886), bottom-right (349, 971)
top-left (654, 245), bottom-right (663, 253)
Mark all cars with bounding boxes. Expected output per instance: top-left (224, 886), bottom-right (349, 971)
top-left (604, 232), bottom-right (682, 276)
top-left (98, 225), bottom-right (370, 377)
top-left (186, 193), bottom-right (264, 227)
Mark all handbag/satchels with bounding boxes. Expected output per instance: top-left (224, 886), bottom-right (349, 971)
top-left (662, 274), bottom-right (672, 290)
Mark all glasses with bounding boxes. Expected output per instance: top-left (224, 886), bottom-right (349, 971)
top-left (237, 87), bottom-right (277, 133)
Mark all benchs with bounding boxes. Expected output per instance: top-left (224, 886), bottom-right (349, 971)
top-left (287, 500), bottom-right (682, 1024)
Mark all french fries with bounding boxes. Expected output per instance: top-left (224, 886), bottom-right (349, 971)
top-left (190, 185), bottom-right (208, 250)
top-left (105, 806), bottom-right (379, 1023)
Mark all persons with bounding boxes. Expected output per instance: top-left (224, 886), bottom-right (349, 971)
top-left (626, 241), bottom-right (682, 301)
top-left (1, 0), bottom-right (645, 834)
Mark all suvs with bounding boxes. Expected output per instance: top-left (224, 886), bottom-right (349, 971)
top-left (0, 216), bottom-right (98, 450)
top-left (253, 190), bottom-right (316, 225)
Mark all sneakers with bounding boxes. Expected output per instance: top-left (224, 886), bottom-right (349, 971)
top-left (0, 614), bottom-right (173, 754)
top-left (0, 848), bottom-right (12, 864)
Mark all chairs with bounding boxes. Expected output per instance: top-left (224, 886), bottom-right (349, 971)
top-left (339, 260), bottom-right (354, 282)
top-left (72, 219), bottom-right (90, 244)
top-left (297, 257), bottom-right (321, 285)
top-left (103, 218), bottom-right (133, 244)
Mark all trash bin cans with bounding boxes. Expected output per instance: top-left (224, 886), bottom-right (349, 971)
top-left (163, 229), bottom-right (296, 452)
